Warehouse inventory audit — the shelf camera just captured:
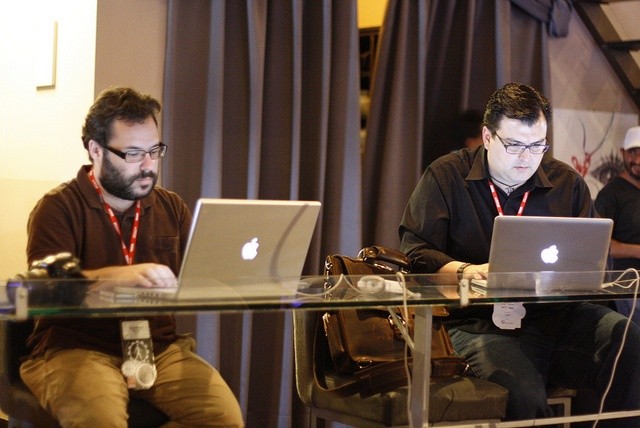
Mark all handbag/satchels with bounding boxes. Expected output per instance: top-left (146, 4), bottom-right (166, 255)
top-left (312, 246), bottom-right (478, 399)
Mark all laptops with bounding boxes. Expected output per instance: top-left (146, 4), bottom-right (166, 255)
top-left (470, 214), bottom-right (615, 293)
top-left (112, 197), bottom-right (323, 299)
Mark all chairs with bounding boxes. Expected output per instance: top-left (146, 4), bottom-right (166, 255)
top-left (0, 285), bottom-right (170, 428)
top-left (292, 275), bottom-right (509, 428)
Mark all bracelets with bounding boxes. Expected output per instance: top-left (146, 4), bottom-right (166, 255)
top-left (456, 262), bottom-right (471, 281)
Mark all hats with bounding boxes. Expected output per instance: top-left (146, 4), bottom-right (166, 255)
top-left (623, 126), bottom-right (640, 152)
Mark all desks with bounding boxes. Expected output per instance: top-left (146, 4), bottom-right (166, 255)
top-left (0, 278), bottom-right (639, 428)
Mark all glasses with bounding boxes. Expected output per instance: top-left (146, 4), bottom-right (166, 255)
top-left (105, 142), bottom-right (168, 163)
top-left (495, 132), bottom-right (551, 154)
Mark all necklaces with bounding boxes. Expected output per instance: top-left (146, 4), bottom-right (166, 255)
top-left (491, 176), bottom-right (518, 195)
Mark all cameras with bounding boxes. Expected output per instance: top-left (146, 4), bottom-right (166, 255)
top-left (6, 252), bottom-right (89, 306)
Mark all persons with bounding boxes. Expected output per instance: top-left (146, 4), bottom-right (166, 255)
top-left (20, 88), bottom-right (245, 427)
top-left (398, 84), bottom-right (640, 427)
top-left (593, 127), bottom-right (639, 321)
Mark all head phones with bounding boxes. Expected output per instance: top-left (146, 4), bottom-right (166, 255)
top-left (356, 275), bottom-right (404, 295)
